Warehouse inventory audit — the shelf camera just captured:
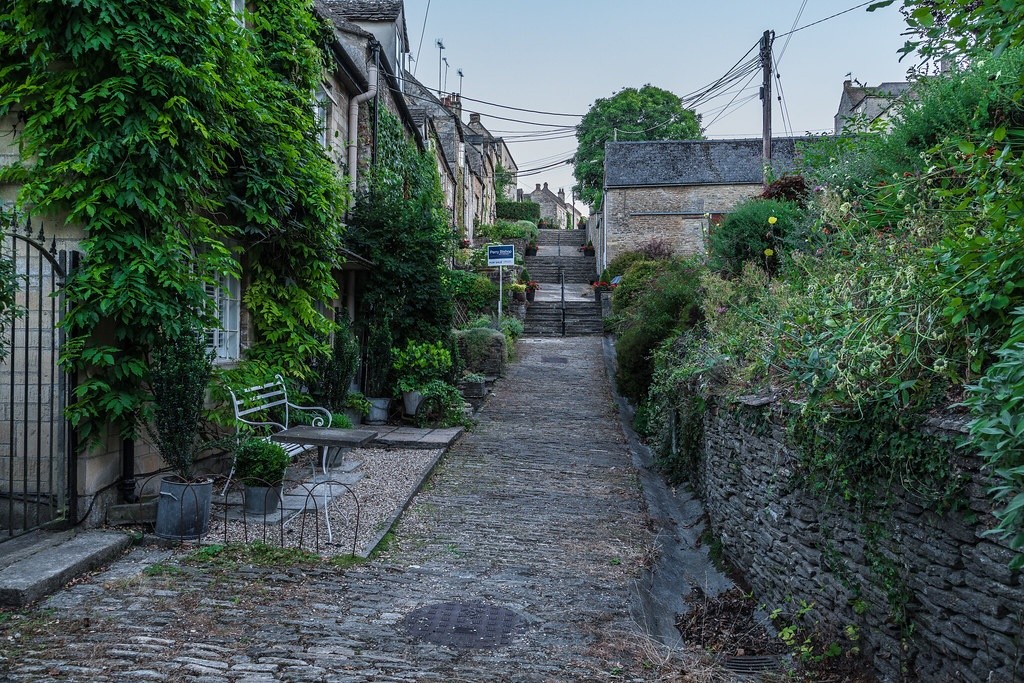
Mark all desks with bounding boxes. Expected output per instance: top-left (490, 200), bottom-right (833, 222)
top-left (271, 426), bottom-right (378, 544)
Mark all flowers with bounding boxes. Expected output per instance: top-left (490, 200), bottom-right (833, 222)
top-left (591, 282), bottom-right (618, 291)
top-left (527, 240), bottom-right (539, 250)
top-left (584, 240), bottom-right (595, 251)
top-left (526, 280), bottom-right (542, 293)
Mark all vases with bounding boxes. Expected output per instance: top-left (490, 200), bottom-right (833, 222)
top-left (584, 250), bottom-right (595, 257)
top-left (526, 249), bottom-right (537, 256)
top-left (595, 291), bottom-right (606, 302)
top-left (526, 291), bottom-right (535, 303)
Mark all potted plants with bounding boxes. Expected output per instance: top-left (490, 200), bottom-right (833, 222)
top-left (577, 217), bottom-right (586, 229)
top-left (510, 283), bottom-right (526, 301)
top-left (132, 328), bottom-right (225, 539)
top-left (391, 338), bottom-right (452, 415)
top-left (364, 378), bottom-right (390, 425)
top-left (538, 219), bottom-right (548, 229)
top-left (233, 438), bottom-right (290, 515)
top-left (461, 372), bottom-right (486, 397)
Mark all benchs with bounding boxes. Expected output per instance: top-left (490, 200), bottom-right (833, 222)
top-left (221, 374), bottom-right (333, 507)
